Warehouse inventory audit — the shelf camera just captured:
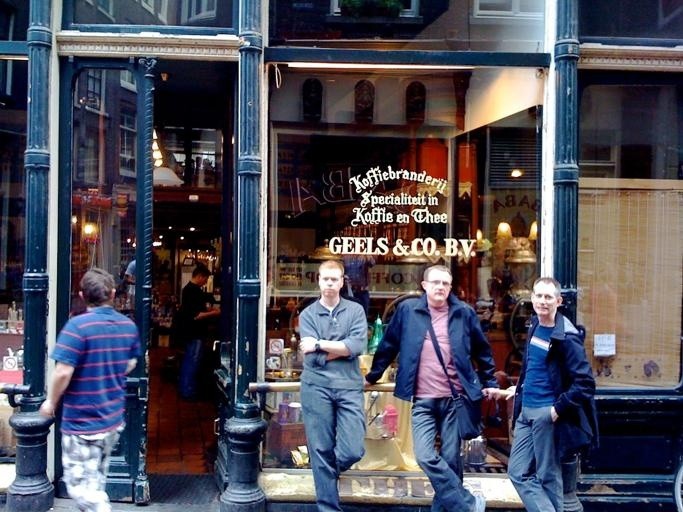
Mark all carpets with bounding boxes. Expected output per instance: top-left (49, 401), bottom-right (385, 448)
top-left (144, 472), bottom-right (220, 505)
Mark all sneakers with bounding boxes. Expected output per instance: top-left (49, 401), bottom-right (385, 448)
top-left (470, 495), bottom-right (487, 512)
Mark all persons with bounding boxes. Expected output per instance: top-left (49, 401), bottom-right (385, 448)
top-left (363, 265), bottom-right (503, 509)
top-left (176, 262), bottom-right (221, 400)
top-left (296, 258), bottom-right (370, 511)
top-left (500, 275), bottom-right (599, 512)
top-left (338, 249), bottom-right (376, 321)
top-left (485, 267), bottom-right (519, 319)
top-left (125, 257), bottom-right (137, 315)
top-left (38, 267), bottom-right (143, 512)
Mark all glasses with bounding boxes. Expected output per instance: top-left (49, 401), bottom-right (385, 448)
top-left (426, 280), bottom-right (453, 289)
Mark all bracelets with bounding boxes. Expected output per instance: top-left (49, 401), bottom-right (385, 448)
top-left (315, 338), bottom-right (321, 353)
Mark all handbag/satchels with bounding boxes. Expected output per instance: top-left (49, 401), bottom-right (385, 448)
top-left (453, 389), bottom-right (486, 442)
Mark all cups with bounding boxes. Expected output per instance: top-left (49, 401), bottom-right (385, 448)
top-left (277, 400), bottom-right (302, 425)
top-left (266, 357), bottom-right (280, 369)
